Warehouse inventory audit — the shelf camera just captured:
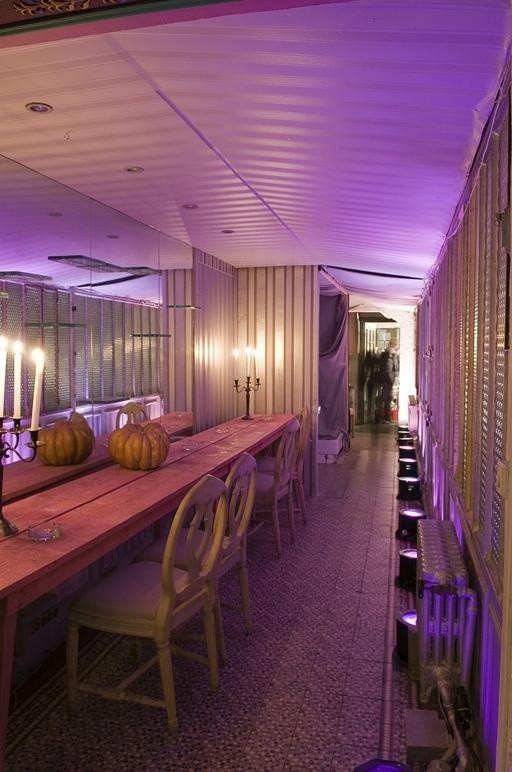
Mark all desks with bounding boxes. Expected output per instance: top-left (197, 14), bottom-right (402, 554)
top-left (1, 414), bottom-right (298, 770)
top-left (0, 410), bottom-right (194, 505)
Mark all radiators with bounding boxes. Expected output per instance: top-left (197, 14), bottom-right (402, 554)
top-left (413, 518), bottom-right (481, 713)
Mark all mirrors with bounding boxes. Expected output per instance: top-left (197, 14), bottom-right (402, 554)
top-left (1, 155), bottom-right (195, 505)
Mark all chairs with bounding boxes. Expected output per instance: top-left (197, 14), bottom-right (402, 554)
top-left (67, 474), bottom-right (231, 740)
top-left (236, 407), bottom-right (308, 561)
top-left (131, 451), bottom-right (259, 672)
top-left (116, 402), bottom-right (149, 429)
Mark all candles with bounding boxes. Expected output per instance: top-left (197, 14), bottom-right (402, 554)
top-left (231, 345), bottom-right (259, 380)
top-left (1, 335), bottom-right (47, 430)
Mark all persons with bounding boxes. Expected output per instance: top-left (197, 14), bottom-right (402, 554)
top-left (374, 336), bottom-right (400, 423)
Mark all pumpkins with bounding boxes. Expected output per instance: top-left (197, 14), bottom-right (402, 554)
top-left (36, 421), bottom-right (95, 465)
top-left (108, 421), bottom-right (171, 471)
